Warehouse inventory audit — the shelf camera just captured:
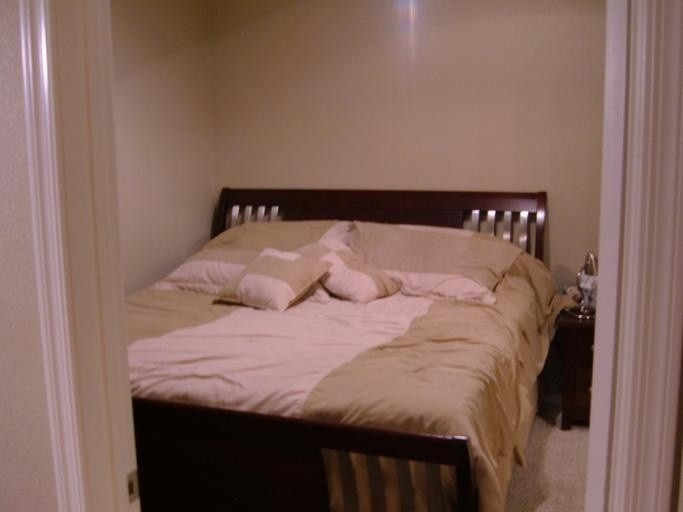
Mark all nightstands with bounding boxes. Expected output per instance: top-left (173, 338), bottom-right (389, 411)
top-left (559, 306), bottom-right (595, 429)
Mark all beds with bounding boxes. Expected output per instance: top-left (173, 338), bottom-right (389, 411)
top-left (124, 188), bottom-right (548, 512)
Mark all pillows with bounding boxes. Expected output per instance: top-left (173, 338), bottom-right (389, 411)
top-left (169, 223), bottom-right (349, 303)
top-left (216, 247), bottom-right (331, 310)
top-left (297, 224), bottom-right (402, 302)
top-left (342, 224), bottom-right (520, 304)
